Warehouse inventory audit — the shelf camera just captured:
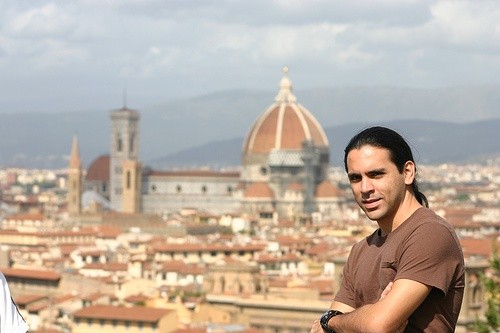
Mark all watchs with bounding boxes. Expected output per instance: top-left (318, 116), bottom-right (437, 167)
top-left (320, 310), bottom-right (344, 333)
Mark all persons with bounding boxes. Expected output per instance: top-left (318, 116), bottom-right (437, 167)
top-left (0, 272), bottom-right (29, 333)
top-left (310, 126), bottom-right (465, 333)
